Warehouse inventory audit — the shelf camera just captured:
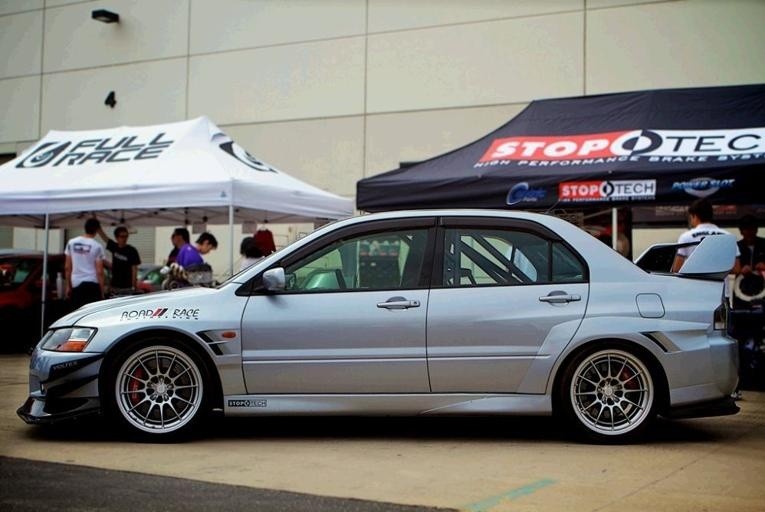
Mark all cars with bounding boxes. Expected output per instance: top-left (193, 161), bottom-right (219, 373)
top-left (14, 205), bottom-right (743, 445)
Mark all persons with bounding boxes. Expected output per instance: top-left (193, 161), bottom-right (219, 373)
top-left (174, 232), bottom-right (217, 288)
top-left (93, 212), bottom-right (142, 298)
top-left (172, 227), bottom-right (189, 249)
top-left (63, 218), bottom-right (105, 314)
top-left (165, 233), bottom-right (180, 266)
top-left (239, 234), bottom-right (268, 271)
top-left (737, 214), bottom-right (765, 276)
top-left (671, 202), bottom-right (741, 273)
